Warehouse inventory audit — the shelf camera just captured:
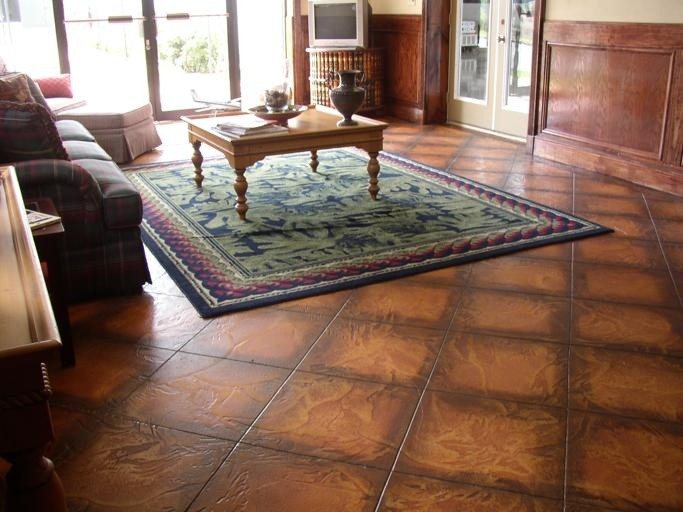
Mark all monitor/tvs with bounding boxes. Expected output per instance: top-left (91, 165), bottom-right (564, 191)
top-left (307, 0), bottom-right (373, 50)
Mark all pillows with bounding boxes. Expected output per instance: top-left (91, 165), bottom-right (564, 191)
top-left (1, 73), bottom-right (35, 103)
top-left (1, 100), bottom-right (68, 161)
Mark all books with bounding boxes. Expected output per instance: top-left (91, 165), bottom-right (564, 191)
top-left (211, 118), bottom-right (290, 141)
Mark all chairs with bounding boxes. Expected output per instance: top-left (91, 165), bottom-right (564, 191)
top-left (0, 57), bottom-right (87, 117)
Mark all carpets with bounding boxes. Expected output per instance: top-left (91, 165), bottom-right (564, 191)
top-left (120, 145), bottom-right (614, 319)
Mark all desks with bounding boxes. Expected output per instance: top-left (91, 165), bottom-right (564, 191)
top-left (180, 104), bottom-right (390, 219)
top-left (305, 47), bottom-right (386, 116)
top-left (0, 165), bottom-right (72, 512)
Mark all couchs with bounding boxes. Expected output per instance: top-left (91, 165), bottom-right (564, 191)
top-left (0, 71), bottom-right (152, 303)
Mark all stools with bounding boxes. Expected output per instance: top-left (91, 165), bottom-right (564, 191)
top-left (57, 104), bottom-right (164, 164)
